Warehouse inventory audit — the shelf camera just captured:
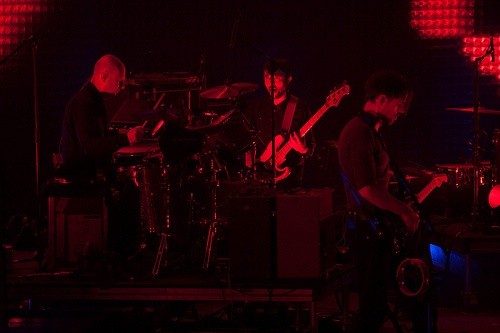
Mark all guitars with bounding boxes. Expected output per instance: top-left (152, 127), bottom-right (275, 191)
top-left (372, 174), bottom-right (449, 246)
top-left (260, 85), bottom-right (350, 184)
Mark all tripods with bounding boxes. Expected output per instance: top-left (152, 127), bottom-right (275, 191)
top-left (123, 128), bottom-right (228, 282)
top-left (456, 97), bottom-right (500, 239)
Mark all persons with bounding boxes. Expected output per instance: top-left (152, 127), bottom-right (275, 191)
top-left (60, 54), bottom-right (146, 250)
top-left (338, 69), bottom-right (422, 332)
top-left (229, 58), bottom-right (316, 191)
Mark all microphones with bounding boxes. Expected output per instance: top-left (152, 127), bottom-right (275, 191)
top-left (263, 54), bottom-right (286, 74)
top-left (490, 33), bottom-right (495, 60)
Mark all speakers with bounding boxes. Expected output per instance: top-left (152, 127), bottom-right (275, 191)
top-left (276, 187), bottom-right (334, 282)
top-left (225, 193), bottom-right (273, 281)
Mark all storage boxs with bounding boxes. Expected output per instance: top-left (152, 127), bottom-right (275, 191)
top-left (275, 188), bottom-right (334, 280)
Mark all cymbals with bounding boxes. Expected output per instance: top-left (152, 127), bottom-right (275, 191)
top-left (200, 81), bottom-right (258, 98)
top-left (447, 107), bottom-right (500, 114)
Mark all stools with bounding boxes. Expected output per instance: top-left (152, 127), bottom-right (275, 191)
top-left (49, 184), bottom-right (106, 273)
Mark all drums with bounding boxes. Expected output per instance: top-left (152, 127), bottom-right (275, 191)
top-left (110, 146), bottom-right (165, 183)
top-left (436, 164), bottom-right (490, 192)
top-left (212, 110), bottom-right (258, 158)
